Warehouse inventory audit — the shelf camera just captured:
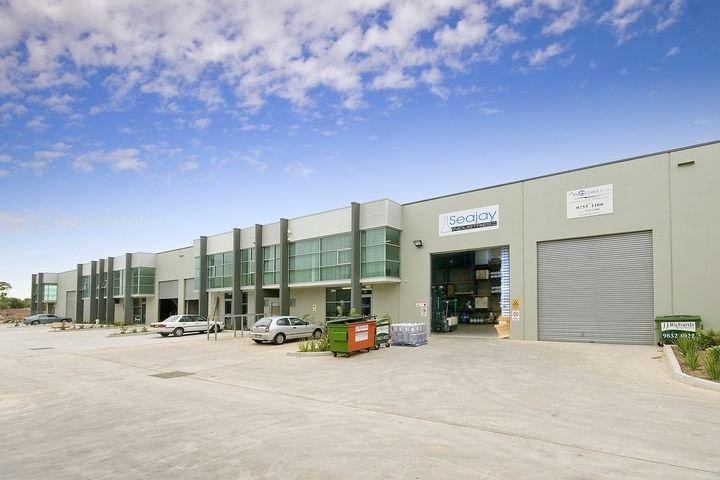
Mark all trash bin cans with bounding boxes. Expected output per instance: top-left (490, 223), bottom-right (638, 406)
top-left (655, 314), bottom-right (703, 347)
top-left (325, 316), bottom-right (390, 357)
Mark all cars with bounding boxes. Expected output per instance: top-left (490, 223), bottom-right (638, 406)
top-left (249, 315), bottom-right (323, 345)
top-left (23, 313), bottom-right (72, 325)
top-left (149, 313), bottom-right (225, 337)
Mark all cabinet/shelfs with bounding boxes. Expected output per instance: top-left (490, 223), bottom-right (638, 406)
top-left (431, 266), bottom-right (501, 324)
